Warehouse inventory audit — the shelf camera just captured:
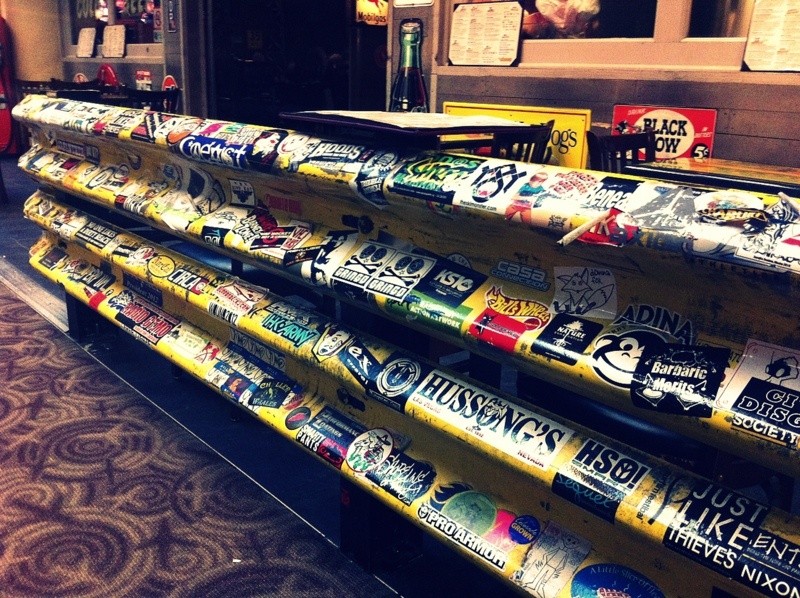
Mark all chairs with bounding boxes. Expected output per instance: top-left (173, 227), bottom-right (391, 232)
top-left (493, 118), bottom-right (555, 163)
top-left (14, 78), bottom-right (181, 153)
top-left (586, 127), bottom-right (657, 173)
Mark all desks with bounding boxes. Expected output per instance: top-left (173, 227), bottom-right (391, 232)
top-left (624, 154), bottom-right (800, 199)
top-left (281, 110), bottom-right (532, 155)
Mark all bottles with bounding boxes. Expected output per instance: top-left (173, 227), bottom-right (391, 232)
top-left (387, 22), bottom-right (429, 114)
